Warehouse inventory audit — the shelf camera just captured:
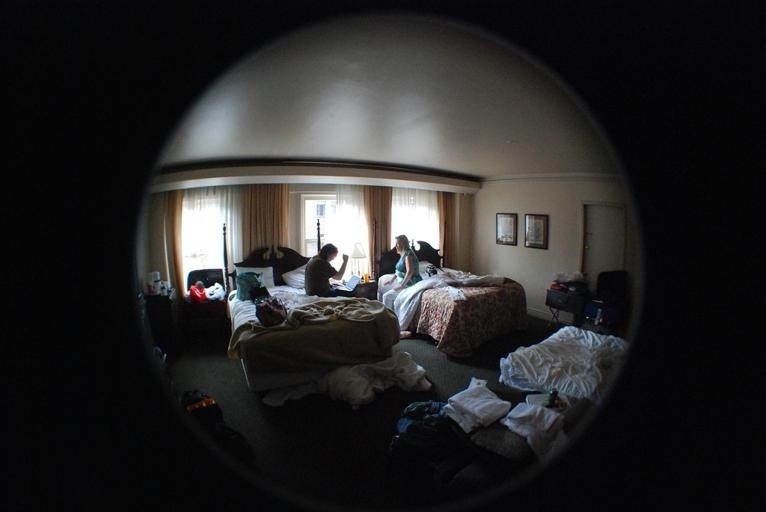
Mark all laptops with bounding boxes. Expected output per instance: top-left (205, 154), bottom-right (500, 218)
top-left (336, 275), bottom-right (360, 292)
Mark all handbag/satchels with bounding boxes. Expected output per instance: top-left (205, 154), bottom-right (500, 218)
top-left (237, 272), bottom-right (286, 325)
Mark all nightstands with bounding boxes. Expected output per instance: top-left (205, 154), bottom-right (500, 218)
top-left (352, 280), bottom-right (375, 298)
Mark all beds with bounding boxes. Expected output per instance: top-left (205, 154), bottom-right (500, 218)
top-left (223, 220), bottom-right (399, 391)
top-left (377, 240), bottom-right (527, 357)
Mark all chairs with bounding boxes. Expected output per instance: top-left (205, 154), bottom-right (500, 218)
top-left (432, 380), bottom-right (591, 463)
top-left (185, 269), bottom-right (226, 344)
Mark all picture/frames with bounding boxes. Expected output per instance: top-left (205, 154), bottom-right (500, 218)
top-left (495, 213), bottom-right (517, 246)
top-left (524, 213), bottom-right (549, 250)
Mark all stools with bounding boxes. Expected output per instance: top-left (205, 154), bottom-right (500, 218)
top-left (497, 325), bottom-right (627, 401)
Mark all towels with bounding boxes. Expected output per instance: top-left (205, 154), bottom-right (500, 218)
top-left (443, 384), bottom-right (558, 445)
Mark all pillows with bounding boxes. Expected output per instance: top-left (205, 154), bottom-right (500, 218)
top-left (235, 265), bottom-right (274, 289)
top-left (418, 260), bottom-right (435, 273)
top-left (280, 262), bottom-right (309, 289)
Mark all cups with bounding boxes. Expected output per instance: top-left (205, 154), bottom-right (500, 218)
top-left (154, 279), bottom-right (161, 294)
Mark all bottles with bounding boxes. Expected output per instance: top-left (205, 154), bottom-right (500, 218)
top-left (363, 272), bottom-right (369, 283)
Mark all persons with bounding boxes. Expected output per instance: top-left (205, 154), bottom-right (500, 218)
top-left (303, 244), bottom-right (413, 340)
top-left (376, 234), bottom-right (423, 308)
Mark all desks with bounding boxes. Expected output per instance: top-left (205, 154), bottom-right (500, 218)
top-left (545, 288), bottom-right (591, 334)
top-left (142, 288), bottom-right (179, 349)
